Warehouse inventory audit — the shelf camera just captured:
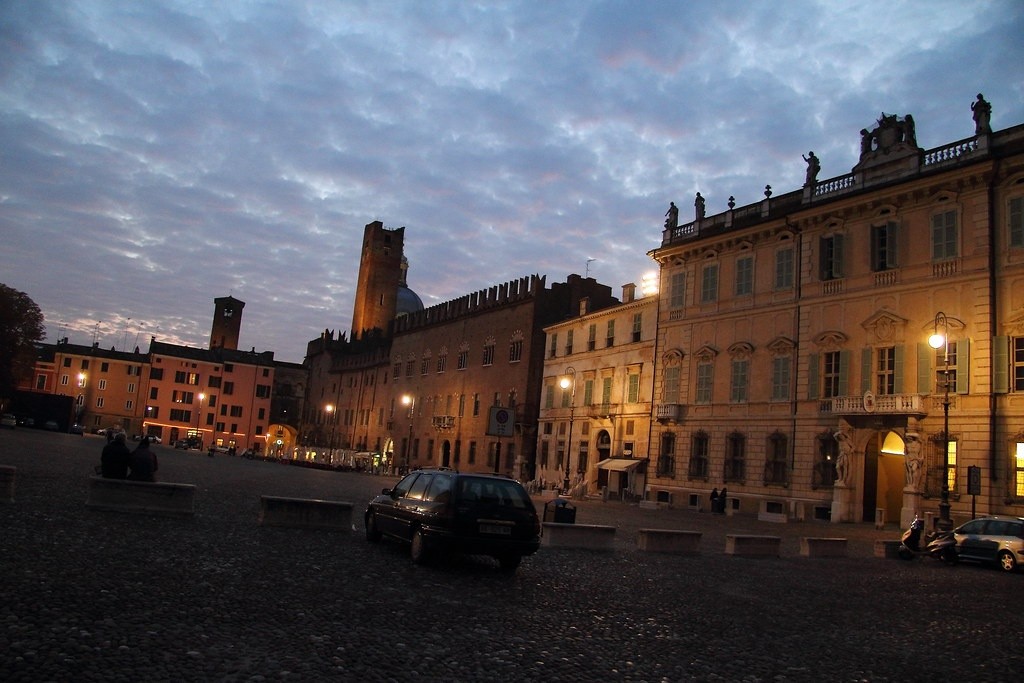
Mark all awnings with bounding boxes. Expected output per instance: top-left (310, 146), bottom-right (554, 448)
top-left (593, 457), bottom-right (646, 487)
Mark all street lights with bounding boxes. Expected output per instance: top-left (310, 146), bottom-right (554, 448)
top-left (326, 399), bottom-right (337, 467)
top-left (559, 366), bottom-right (577, 498)
top-left (928, 311), bottom-right (954, 530)
top-left (401, 391), bottom-right (416, 480)
top-left (196, 389), bottom-right (206, 438)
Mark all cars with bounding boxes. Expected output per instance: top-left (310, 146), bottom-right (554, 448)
top-left (71, 424), bottom-right (83, 435)
top-left (0, 413), bottom-right (17, 430)
top-left (97, 426), bottom-right (125, 436)
top-left (19, 417), bottom-right (37, 428)
top-left (45, 421), bottom-right (59, 431)
top-left (215, 445), bottom-right (229, 454)
top-left (174, 438), bottom-right (188, 450)
top-left (952, 515), bottom-right (1024, 572)
top-left (135, 435), bottom-right (162, 444)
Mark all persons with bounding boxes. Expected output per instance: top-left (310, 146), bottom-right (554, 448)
top-left (505, 471), bottom-right (512, 478)
top-left (665, 202), bottom-right (678, 226)
top-left (101, 433), bottom-right (133, 480)
top-left (860, 130), bottom-right (873, 158)
top-left (802, 151), bottom-right (820, 185)
top-left (127, 439), bottom-right (158, 482)
top-left (104, 428), bottom-right (115, 444)
top-left (710, 488), bottom-right (728, 515)
top-left (229, 445), bottom-right (237, 456)
top-left (190, 435), bottom-right (195, 448)
top-left (210, 441), bottom-right (216, 456)
top-left (901, 115), bottom-right (918, 149)
top-left (133, 434), bottom-right (151, 440)
top-left (694, 192), bottom-right (705, 217)
top-left (903, 436), bottom-right (922, 488)
top-left (971, 93), bottom-right (993, 134)
top-left (833, 431), bottom-right (853, 484)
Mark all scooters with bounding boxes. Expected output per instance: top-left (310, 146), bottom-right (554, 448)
top-left (896, 514), bottom-right (959, 565)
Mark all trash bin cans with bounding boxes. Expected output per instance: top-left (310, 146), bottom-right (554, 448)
top-left (541, 498), bottom-right (576, 537)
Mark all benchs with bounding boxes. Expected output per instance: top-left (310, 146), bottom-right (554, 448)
top-left (84, 474), bottom-right (197, 516)
top-left (799, 536), bottom-right (849, 558)
top-left (542, 522), bottom-right (616, 548)
top-left (638, 528), bottom-right (703, 552)
top-left (873, 540), bottom-right (903, 558)
top-left (260, 495), bottom-right (353, 530)
top-left (725, 534), bottom-right (781, 556)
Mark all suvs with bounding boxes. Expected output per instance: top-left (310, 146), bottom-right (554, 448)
top-left (364, 466), bottom-right (543, 570)
